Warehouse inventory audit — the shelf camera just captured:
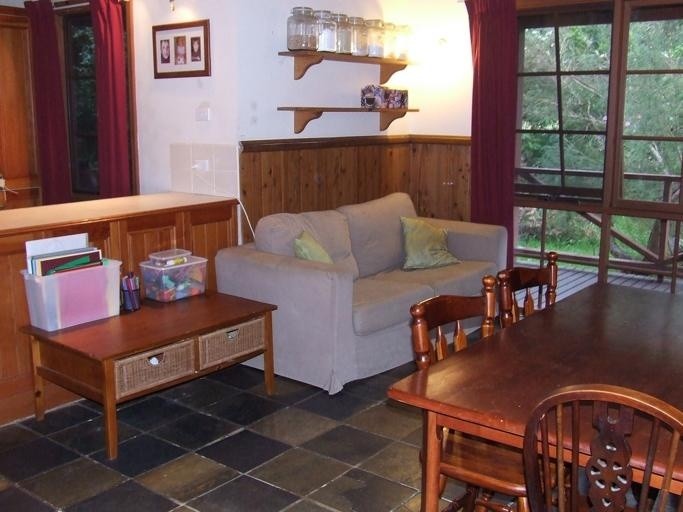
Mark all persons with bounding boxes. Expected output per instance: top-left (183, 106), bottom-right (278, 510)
top-left (174, 37), bottom-right (186, 64)
top-left (161, 40), bottom-right (170, 63)
top-left (192, 38), bottom-right (201, 61)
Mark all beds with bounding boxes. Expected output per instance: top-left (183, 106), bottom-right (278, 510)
top-left (212, 192), bottom-right (510, 398)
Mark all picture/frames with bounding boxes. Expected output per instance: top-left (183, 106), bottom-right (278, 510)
top-left (149, 18), bottom-right (211, 80)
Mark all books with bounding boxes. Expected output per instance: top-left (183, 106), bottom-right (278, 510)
top-left (25, 233), bottom-right (103, 276)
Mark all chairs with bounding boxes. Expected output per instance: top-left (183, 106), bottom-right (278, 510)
top-left (495, 251), bottom-right (561, 326)
top-left (521, 383), bottom-right (683, 512)
top-left (408, 275), bottom-right (571, 512)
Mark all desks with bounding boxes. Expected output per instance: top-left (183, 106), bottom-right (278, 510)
top-left (385, 283), bottom-right (682, 507)
top-left (18, 289), bottom-right (277, 460)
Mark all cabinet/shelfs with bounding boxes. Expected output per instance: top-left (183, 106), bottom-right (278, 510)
top-left (282, 53), bottom-right (422, 131)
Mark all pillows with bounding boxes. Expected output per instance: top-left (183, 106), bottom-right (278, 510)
top-left (292, 231), bottom-right (334, 266)
top-left (396, 215), bottom-right (463, 270)
top-left (342, 191), bottom-right (417, 284)
top-left (254, 210), bottom-right (358, 278)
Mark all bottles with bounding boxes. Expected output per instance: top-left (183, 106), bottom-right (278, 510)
top-left (287, 7), bottom-right (415, 60)
